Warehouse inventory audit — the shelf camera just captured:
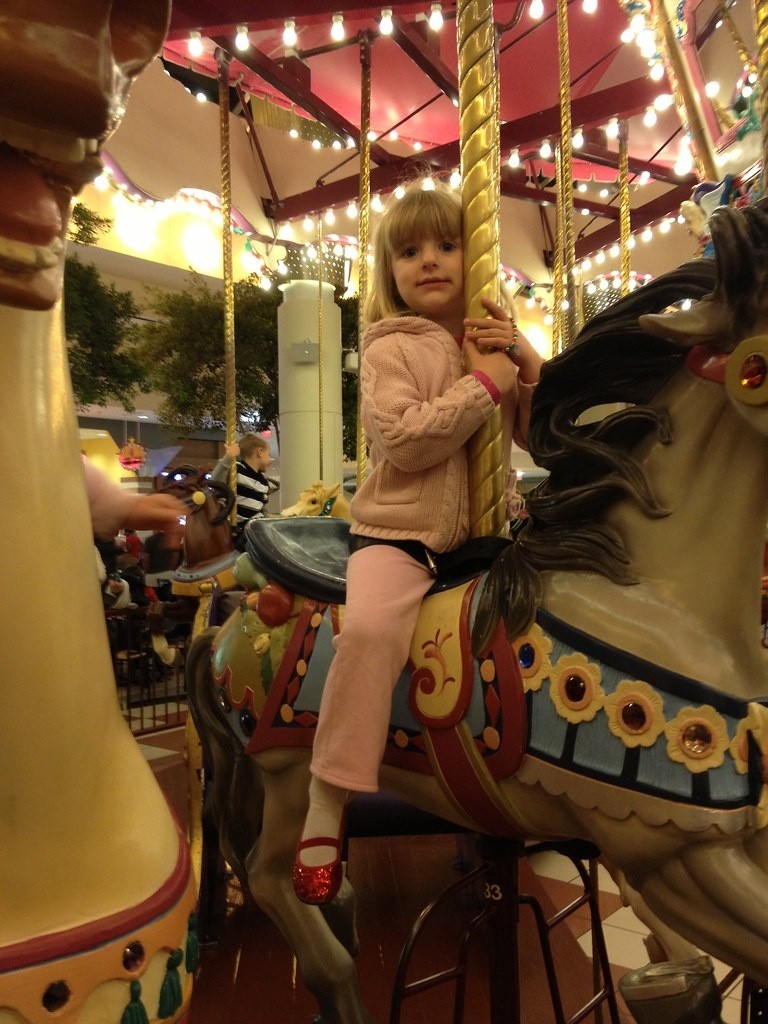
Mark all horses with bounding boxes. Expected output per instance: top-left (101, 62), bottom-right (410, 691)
top-left (0, 0), bottom-right (767, 1024)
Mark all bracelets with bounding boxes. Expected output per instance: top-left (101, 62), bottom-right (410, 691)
top-left (500, 316), bottom-right (518, 352)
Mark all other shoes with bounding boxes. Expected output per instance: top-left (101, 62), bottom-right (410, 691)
top-left (291, 811), bottom-right (346, 906)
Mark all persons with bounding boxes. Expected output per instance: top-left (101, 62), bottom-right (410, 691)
top-left (210, 432), bottom-right (274, 550)
top-left (79, 455), bottom-right (192, 605)
top-left (291, 187), bottom-right (547, 899)
